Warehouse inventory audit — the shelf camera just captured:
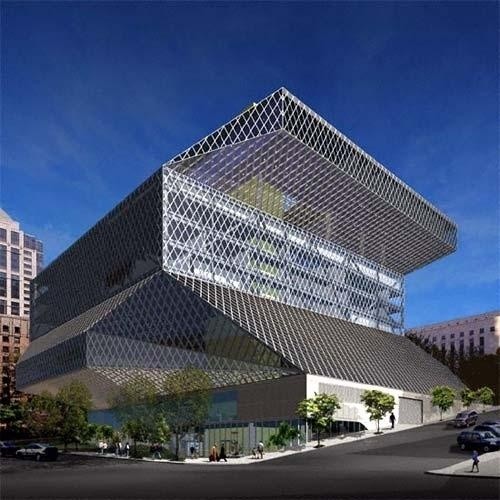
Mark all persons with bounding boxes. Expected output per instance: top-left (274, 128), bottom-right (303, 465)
top-left (190, 445), bottom-right (195, 460)
top-left (95, 440), bottom-right (130, 456)
top-left (390, 413), bottom-right (395, 428)
top-left (210, 442), bottom-right (218, 463)
top-left (216, 443), bottom-right (227, 462)
top-left (468, 449), bottom-right (479, 472)
top-left (258, 440), bottom-right (264, 459)
top-left (155, 442), bottom-right (162, 461)
top-left (194, 445), bottom-right (200, 459)
top-left (149, 442), bottom-right (156, 460)
